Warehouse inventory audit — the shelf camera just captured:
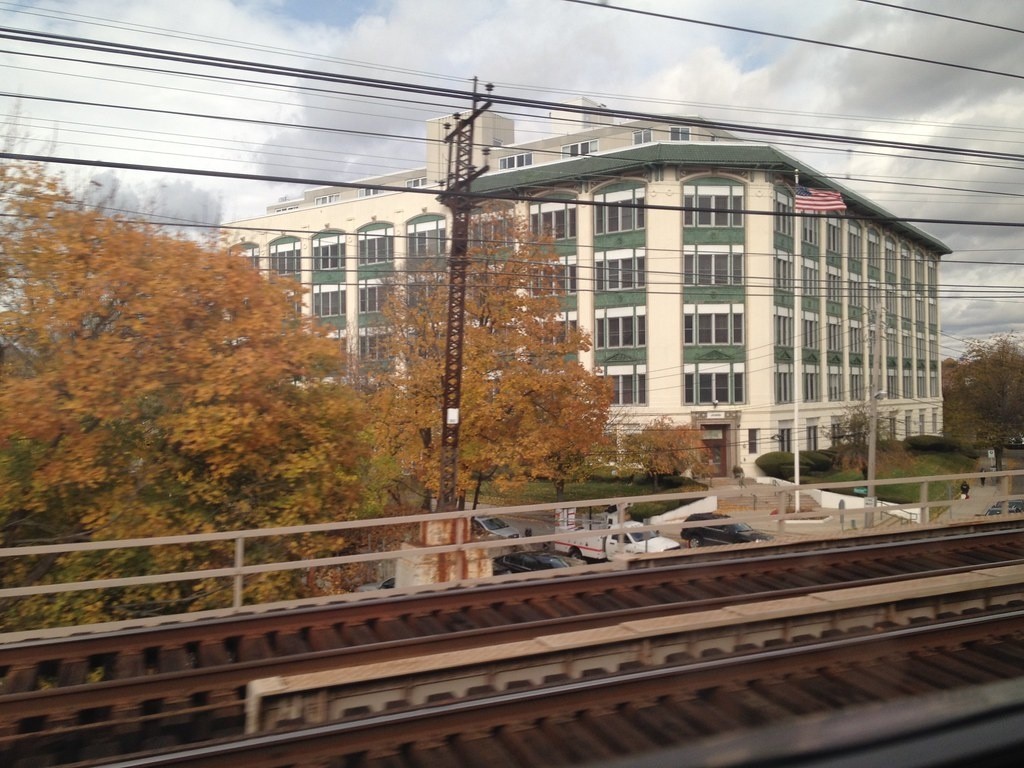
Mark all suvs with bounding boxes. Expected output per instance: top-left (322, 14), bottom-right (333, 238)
top-left (681, 513), bottom-right (774, 550)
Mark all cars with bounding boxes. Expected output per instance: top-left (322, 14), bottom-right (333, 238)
top-left (472, 517), bottom-right (520, 539)
top-left (355, 575), bottom-right (396, 592)
top-left (492, 550), bottom-right (569, 576)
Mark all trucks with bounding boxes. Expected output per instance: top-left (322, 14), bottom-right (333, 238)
top-left (554, 521), bottom-right (681, 564)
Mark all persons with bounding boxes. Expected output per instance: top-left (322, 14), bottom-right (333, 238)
top-left (961, 481), bottom-right (969, 495)
top-left (980, 468), bottom-right (986, 486)
